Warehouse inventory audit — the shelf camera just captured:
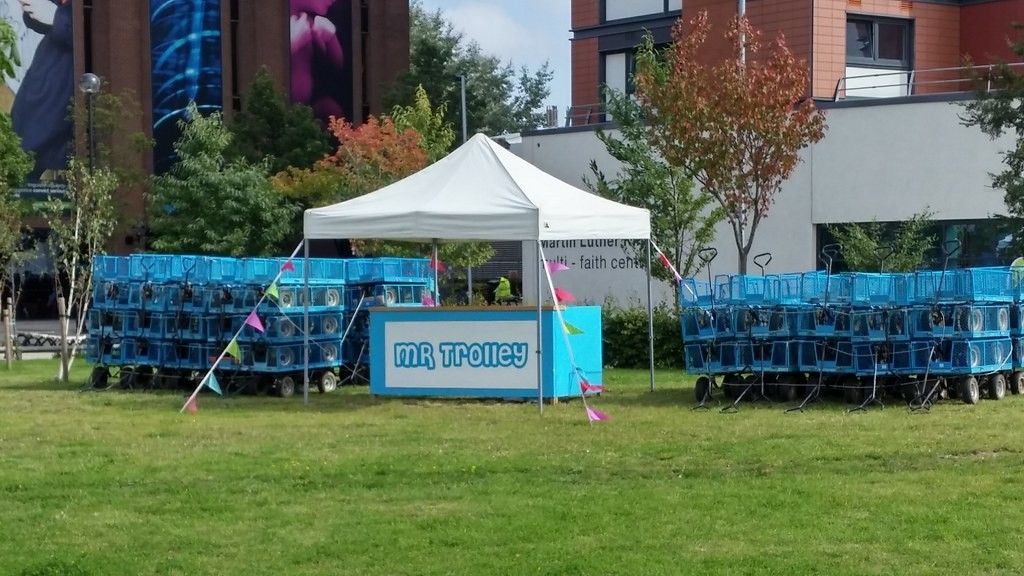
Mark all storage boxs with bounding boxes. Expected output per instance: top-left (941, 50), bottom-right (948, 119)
top-left (86, 253), bottom-right (441, 375)
top-left (679, 265), bottom-right (1024, 378)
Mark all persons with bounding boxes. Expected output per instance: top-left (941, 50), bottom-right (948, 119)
top-left (502, 269), bottom-right (521, 297)
top-left (290, 0), bottom-right (345, 121)
top-left (1006, 246), bottom-right (1024, 294)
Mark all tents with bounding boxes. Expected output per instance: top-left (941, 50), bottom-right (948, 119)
top-left (303, 133), bottom-right (655, 413)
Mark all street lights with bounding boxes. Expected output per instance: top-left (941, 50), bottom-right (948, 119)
top-left (76, 73), bottom-right (103, 179)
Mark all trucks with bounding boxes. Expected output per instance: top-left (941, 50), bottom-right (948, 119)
top-left (0, 175), bottom-right (82, 318)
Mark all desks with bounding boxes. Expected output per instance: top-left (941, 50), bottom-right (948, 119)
top-left (366, 305), bottom-right (604, 404)
